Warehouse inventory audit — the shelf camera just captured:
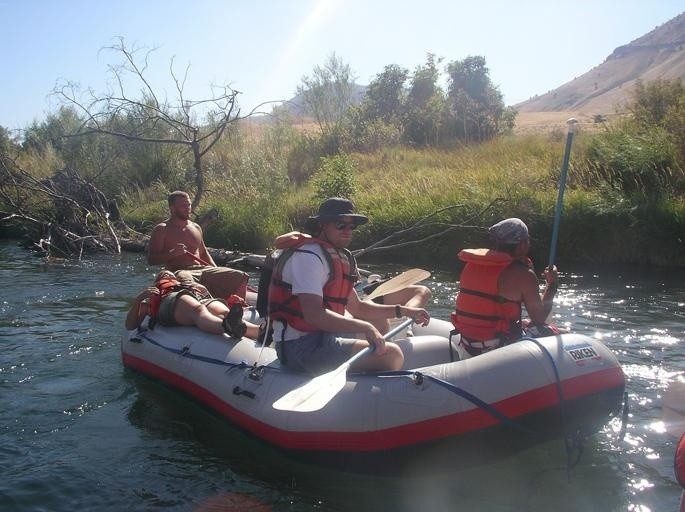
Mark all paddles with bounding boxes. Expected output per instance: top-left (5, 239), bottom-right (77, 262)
top-left (542, 118), bottom-right (577, 326)
top-left (362, 268), bottom-right (430, 305)
top-left (273, 318), bottom-right (413, 411)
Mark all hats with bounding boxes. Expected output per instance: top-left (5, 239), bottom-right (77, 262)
top-left (306, 198), bottom-right (368, 224)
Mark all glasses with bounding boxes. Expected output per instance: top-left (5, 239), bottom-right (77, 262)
top-left (329, 220), bottom-right (358, 229)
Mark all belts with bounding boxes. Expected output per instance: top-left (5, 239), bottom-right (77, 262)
top-left (462, 337), bottom-right (501, 348)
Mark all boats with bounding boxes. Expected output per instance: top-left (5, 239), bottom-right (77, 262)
top-left (121, 288), bottom-right (632, 453)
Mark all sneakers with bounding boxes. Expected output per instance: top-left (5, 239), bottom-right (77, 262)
top-left (222, 302), bottom-right (246, 339)
top-left (400, 316), bottom-right (413, 337)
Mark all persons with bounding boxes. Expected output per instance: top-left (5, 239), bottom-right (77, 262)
top-left (147, 190), bottom-right (252, 306)
top-left (353, 273), bottom-right (434, 338)
top-left (269, 198), bottom-right (432, 374)
top-left (125, 268), bottom-right (273, 346)
top-left (450, 218), bottom-right (571, 356)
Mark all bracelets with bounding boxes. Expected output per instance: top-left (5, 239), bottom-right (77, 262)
top-left (393, 304), bottom-right (402, 321)
